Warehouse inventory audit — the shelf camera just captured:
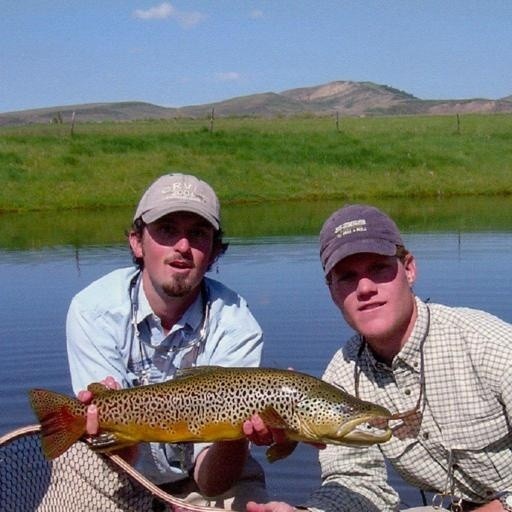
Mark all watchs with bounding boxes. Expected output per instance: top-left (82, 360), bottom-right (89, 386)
top-left (489, 491), bottom-right (512, 512)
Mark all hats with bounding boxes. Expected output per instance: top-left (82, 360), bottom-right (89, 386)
top-left (318, 204), bottom-right (407, 275)
top-left (131, 172), bottom-right (223, 234)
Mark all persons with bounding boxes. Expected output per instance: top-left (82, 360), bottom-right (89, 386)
top-left (245, 205), bottom-right (512, 512)
top-left (34, 171), bottom-right (327, 512)
